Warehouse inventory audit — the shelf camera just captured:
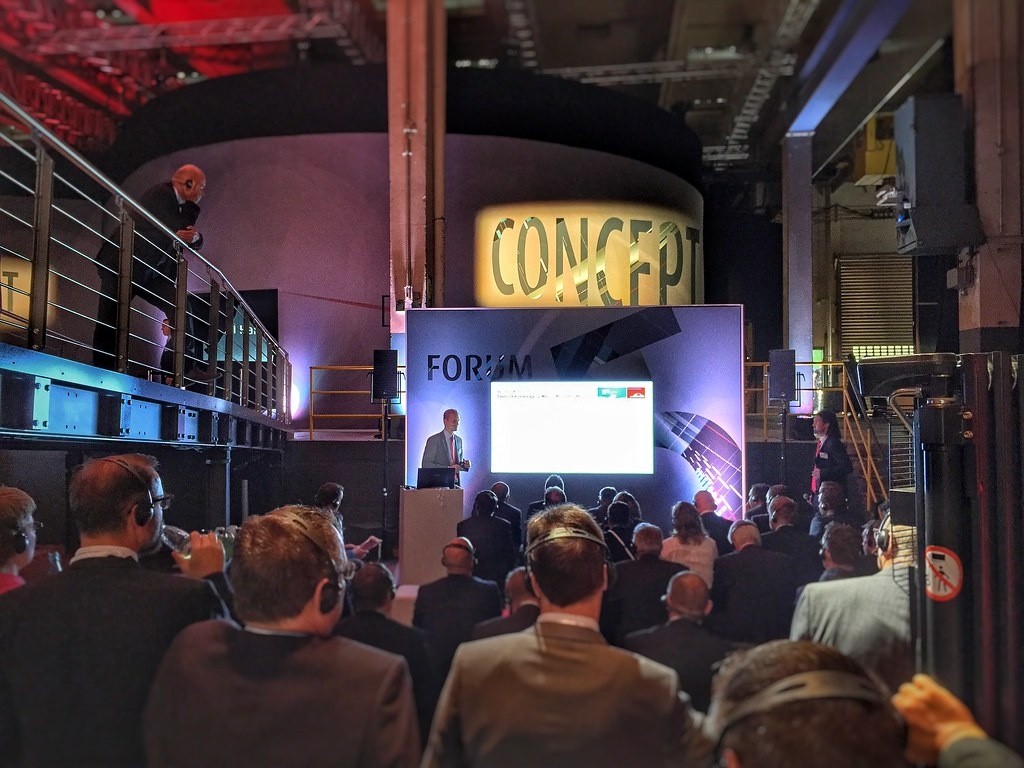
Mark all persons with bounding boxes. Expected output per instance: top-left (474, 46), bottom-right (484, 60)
top-left (90, 159), bottom-right (224, 387)
top-left (159, 317), bottom-right (174, 385)
top-left (0, 406), bottom-right (1024, 768)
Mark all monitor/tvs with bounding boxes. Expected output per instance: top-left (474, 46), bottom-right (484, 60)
top-left (190, 287), bottom-right (280, 362)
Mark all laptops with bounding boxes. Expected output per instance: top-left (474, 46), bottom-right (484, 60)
top-left (416, 468), bottom-right (456, 491)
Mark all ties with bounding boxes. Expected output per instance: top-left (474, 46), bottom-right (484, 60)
top-left (178, 204), bottom-right (185, 232)
top-left (812, 438), bottom-right (822, 494)
top-left (449, 435), bottom-right (458, 482)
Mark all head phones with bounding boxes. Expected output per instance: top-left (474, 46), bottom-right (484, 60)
top-left (874, 514), bottom-right (891, 551)
top-left (771, 501), bottom-right (797, 524)
top-left (439, 543), bottom-right (480, 570)
top-left (821, 493), bottom-right (830, 511)
top-left (691, 498), bottom-right (697, 509)
top-left (349, 564), bottom-right (397, 605)
top-left (866, 520), bottom-right (879, 548)
top-left (596, 494), bottom-right (614, 504)
top-left (658, 594), bottom-right (713, 625)
top-left (4, 517), bottom-right (30, 555)
top-left (629, 537), bottom-right (638, 550)
top-left (748, 493), bottom-right (763, 502)
top-left (269, 508), bottom-right (344, 616)
top-left (171, 177), bottom-right (192, 189)
top-left (601, 511), bottom-right (614, 523)
top-left (312, 482), bottom-right (343, 508)
top-left (818, 529), bottom-right (836, 559)
top-left (541, 501), bottom-right (548, 509)
top-left (522, 528), bottom-right (618, 598)
top-left (100, 456), bottom-right (156, 525)
top-left (728, 520), bottom-right (757, 548)
top-left (701, 668), bottom-right (908, 768)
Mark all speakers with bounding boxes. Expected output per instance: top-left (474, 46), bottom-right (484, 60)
top-left (767, 349), bottom-right (796, 404)
top-left (372, 349), bottom-right (398, 398)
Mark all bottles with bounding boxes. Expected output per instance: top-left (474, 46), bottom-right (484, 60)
top-left (47, 550), bottom-right (63, 579)
top-left (158, 523), bottom-right (194, 560)
top-left (214, 524), bottom-right (239, 562)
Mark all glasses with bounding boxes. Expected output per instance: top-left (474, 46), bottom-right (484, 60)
top-left (23, 521), bottom-right (44, 534)
top-left (338, 562), bottom-right (358, 580)
top-left (152, 490), bottom-right (175, 509)
